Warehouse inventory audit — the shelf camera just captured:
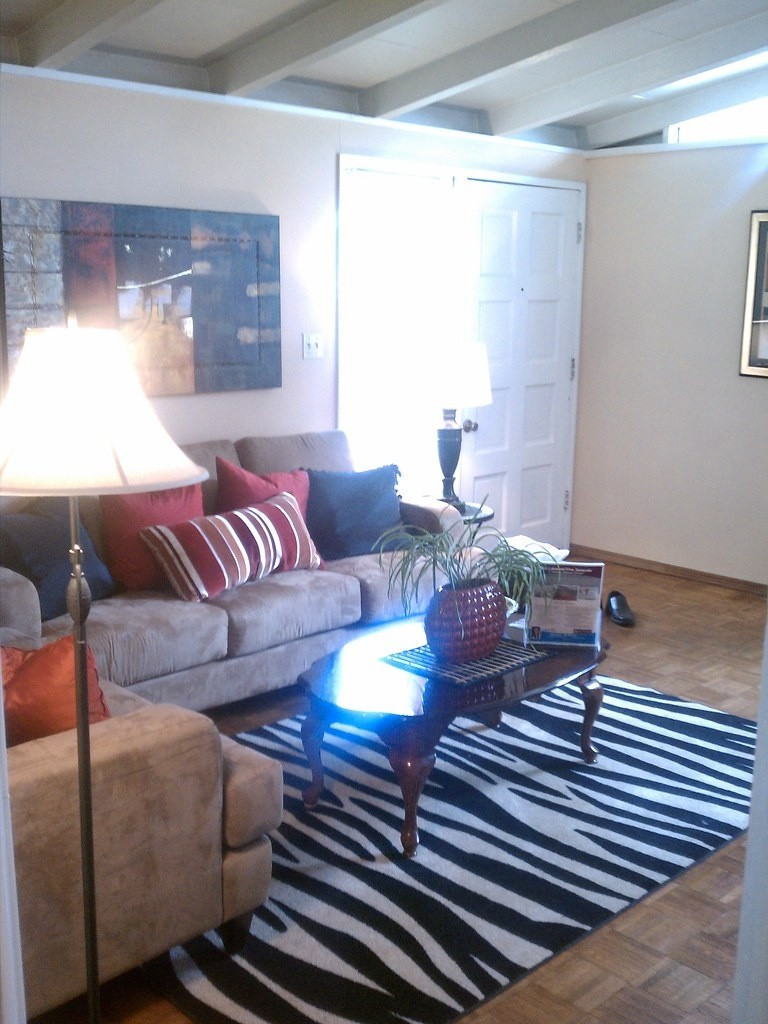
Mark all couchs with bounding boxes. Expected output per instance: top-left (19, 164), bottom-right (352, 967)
top-left (0, 429), bottom-right (492, 1024)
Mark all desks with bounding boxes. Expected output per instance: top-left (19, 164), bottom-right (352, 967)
top-left (296, 618), bottom-right (610, 859)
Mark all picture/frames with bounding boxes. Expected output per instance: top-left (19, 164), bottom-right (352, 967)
top-left (739, 210), bottom-right (768, 378)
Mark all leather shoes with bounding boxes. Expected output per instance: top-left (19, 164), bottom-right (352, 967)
top-left (605, 591), bottom-right (635, 628)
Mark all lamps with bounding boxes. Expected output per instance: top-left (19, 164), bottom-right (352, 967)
top-left (410, 348), bottom-right (494, 516)
top-left (0, 326), bottom-right (212, 1024)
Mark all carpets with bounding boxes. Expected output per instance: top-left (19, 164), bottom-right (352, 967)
top-left (124, 675), bottom-right (756, 1024)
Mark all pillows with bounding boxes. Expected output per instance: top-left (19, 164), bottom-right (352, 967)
top-left (0, 497), bottom-right (118, 623)
top-left (0, 634), bottom-right (113, 748)
top-left (215, 455), bottom-right (328, 572)
top-left (99, 482), bottom-right (205, 592)
top-left (305, 463), bottom-right (420, 562)
top-left (136, 488), bottom-right (328, 604)
